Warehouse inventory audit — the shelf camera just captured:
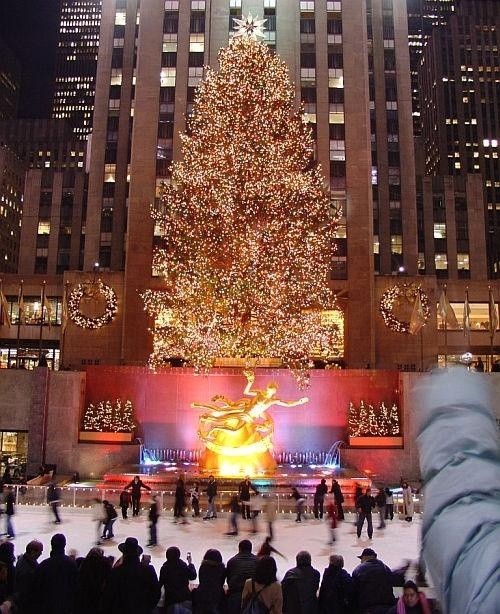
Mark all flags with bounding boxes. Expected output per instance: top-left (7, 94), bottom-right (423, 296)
top-left (0, 294), bottom-right (12, 330)
top-left (18, 285), bottom-right (25, 326)
top-left (62, 286), bottom-right (68, 338)
top-left (405, 292), bottom-right (427, 338)
top-left (434, 288), bottom-right (459, 331)
top-left (486, 288), bottom-right (500, 340)
top-left (41, 287), bottom-right (53, 332)
top-left (462, 296), bottom-right (473, 335)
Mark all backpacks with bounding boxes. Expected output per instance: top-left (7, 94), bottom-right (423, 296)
top-left (241, 578), bottom-right (270, 614)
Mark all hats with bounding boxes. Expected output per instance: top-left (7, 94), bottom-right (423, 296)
top-left (358, 549), bottom-right (377, 558)
top-left (118, 537), bottom-right (143, 556)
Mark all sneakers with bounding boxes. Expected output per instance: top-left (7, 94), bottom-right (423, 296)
top-left (242, 517), bottom-right (252, 519)
top-left (405, 517), bottom-right (412, 521)
top-left (55, 520), bottom-right (60, 522)
top-left (172, 521), bottom-right (187, 524)
top-left (202, 516), bottom-right (217, 519)
top-left (133, 512), bottom-right (138, 516)
top-left (358, 535), bottom-right (360, 538)
top-left (378, 527), bottom-right (385, 529)
top-left (227, 532), bottom-right (238, 535)
top-left (315, 515), bottom-right (322, 518)
top-left (369, 535), bottom-right (372, 538)
top-left (100, 535), bottom-right (110, 540)
top-left (147, 539), bottom-right (155, 546)
top-left (295, 519), bottom-right (301, 521)
top-left (123, 515), bottom-right (127, 518)
top-left (4, 533), bottom-right (14, 538)
top-left (384, 516), bottom-right (393, 520)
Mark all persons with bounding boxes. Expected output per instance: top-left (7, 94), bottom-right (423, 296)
top-left (0, 533), bottom-right (435, 614)
top-left (170, 475), bottom-right (218, 525)
top-left (223, 474), bottom-right (277, 540)
top-left (46, 484), bottom-right (63, 524)
top-left (90, 496), bottom-right (118, 547)
top-left (0, 453), bottom-right (19, 539)
top-left (352, 476), bottom-right (414, 540)
top-left (313, 478), bottom-right (345, 520)
top-left (147, 495), bottom-right (160, 548)
top-left (474, 356), bottom-right (484, 372)
top-left (288, 488), bottom-right (306, 523)
top-left (119, 474), bottom-right (152, 520)
top-left (191, 370), bottom-right (309, 432)
top-left (323, 494), bottom-right (338, 545)
